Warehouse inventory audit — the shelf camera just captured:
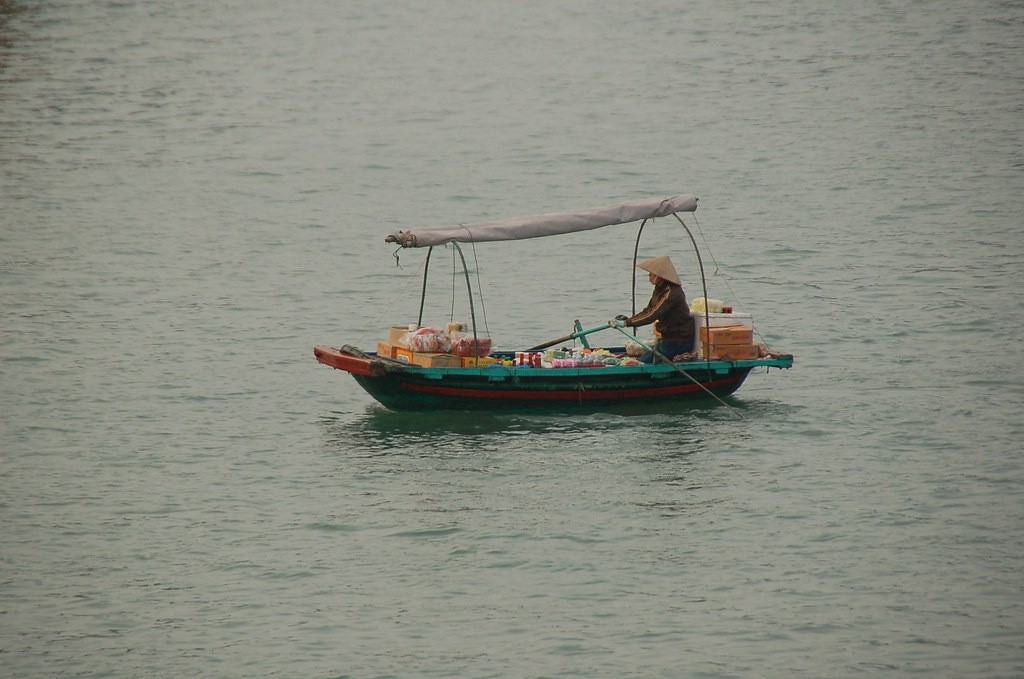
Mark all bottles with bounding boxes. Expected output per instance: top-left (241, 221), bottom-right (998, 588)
top-left (498, 349), bottom-right (601, 368)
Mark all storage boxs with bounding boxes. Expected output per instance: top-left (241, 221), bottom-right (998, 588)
top-left (699, 322), bottom-right (758, 360)
top-left (377, 326), bottom-right (498, 368)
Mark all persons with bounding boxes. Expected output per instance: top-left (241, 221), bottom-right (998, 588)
top-left (608, 256), bottom-right (695, 364)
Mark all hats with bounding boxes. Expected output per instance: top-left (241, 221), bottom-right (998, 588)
top-left (639, 255), bottom-right (681, 285)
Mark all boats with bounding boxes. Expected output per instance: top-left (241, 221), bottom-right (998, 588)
top-left (313, 194), bottom-right (793, 414)
